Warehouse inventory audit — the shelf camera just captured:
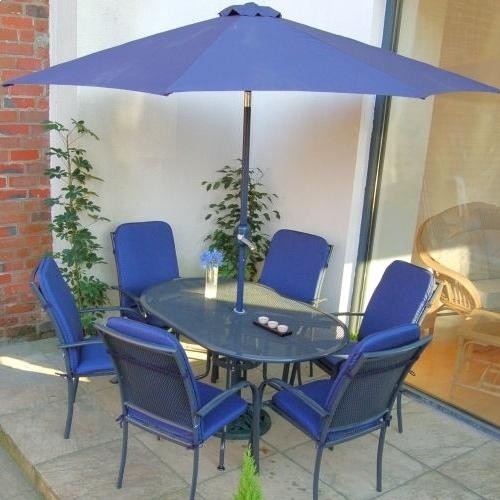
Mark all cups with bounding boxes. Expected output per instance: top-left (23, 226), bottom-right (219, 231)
top-left (258, 316), bottom-right (289, 336)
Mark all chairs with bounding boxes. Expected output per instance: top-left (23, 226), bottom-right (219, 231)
top-left (286, 262), bottom-right (436, 453)
top-left (33, 252), bottom-right (162, 442)
top-left (416, 199), bottom-right (500, 339)
top-left (93, 317), bottom-right (262, 500)
top-left (213, 229), bottom-right (332, 383)
top-left (112, 221), bottom-right (209, 378)
top-left (255, 322), bottom-right (432, 500)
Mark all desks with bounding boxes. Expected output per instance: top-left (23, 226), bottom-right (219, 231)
top-left (140, 278), bottom-right (345, 441)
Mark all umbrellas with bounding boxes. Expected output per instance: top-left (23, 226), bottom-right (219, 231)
top-left (0, 1), bottom-right (500, 312)
